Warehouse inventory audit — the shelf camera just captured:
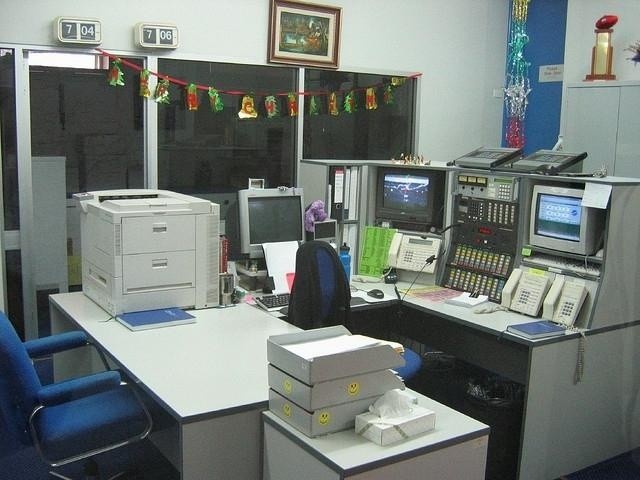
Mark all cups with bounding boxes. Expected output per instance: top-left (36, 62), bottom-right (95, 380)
top-left (220, 272), bottom-right (235, 305)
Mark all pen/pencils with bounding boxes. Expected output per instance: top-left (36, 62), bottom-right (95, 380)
top-left (555, 174), bottom-right (594, 177)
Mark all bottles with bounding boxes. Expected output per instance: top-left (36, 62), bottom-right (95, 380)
top-left (340, 242), bottom-right (351, 286)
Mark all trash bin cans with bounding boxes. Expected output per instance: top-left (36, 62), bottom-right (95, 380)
top-left (463, 379), bottom-right (523, 480)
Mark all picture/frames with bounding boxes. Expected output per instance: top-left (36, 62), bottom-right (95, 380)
top-left (265, 0), bottom-right (343, 70)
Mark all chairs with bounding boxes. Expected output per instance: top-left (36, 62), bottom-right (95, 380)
top-left (283, 240), bottom-right (425, 384)
top-left (0, 306), bottom-right (175, 480)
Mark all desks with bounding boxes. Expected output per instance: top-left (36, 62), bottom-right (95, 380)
top-left (257, 380), bottom-right (490, 479)
top-left (393, 272), bottom-right (640, 478)
top-left (39, 281), bottom-right (314, 479)
top-left (222, 258), bottom-right (398, 327)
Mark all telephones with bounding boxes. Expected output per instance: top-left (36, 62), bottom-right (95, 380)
top-left (500, 268), bottom-right (552, 317)
top-left (542, 278), bottom-right (590, 327)
top-left (388, 232), bottom-right (443, 274)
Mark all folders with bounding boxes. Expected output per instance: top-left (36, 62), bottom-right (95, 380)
top-left (328, 167), bottom-right (344, 219)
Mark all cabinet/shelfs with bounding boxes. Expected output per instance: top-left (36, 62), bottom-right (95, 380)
top-left (557, 78), bottom-right (640, 180)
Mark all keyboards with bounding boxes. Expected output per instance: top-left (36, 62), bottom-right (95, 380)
top-left (251, 291), bottom-right (290, 312)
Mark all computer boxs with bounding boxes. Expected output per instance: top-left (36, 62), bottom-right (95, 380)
top-left (305, 218), bottom-right (339, 253)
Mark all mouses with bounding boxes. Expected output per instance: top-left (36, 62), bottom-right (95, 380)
top-left (596, 248), bottom-right (604, 258)
top-left (367, 289), bottom-right (384, 299)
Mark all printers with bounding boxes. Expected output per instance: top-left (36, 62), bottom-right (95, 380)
top-left (72, 189), bottom-right (220, 319)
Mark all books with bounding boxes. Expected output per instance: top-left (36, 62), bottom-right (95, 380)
top-left (507, 320), bottom-right (566, 340)
top-left (115, 306), bottom-right (199, 332)
top-left (330, 165), bottom-right (365, 275)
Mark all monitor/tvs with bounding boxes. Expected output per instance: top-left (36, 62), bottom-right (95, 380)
top-left (224, 188), bottom-right (305, 260)
top-left (530, 184), bottom-right (606, 256)
top-left (374, 167), bottom-right (446, 232)
